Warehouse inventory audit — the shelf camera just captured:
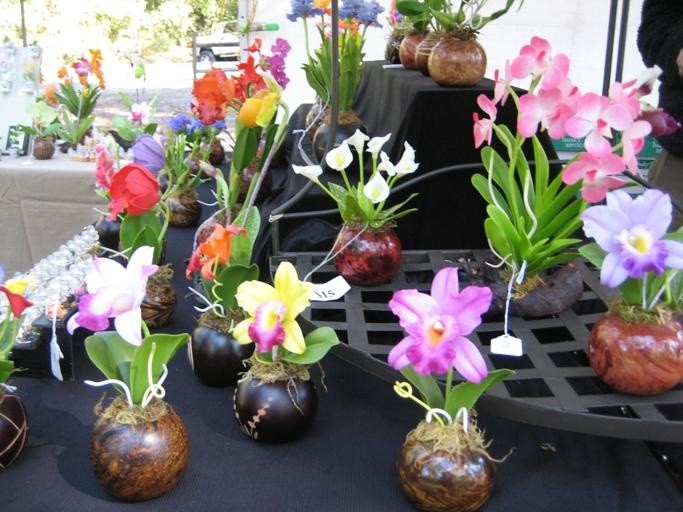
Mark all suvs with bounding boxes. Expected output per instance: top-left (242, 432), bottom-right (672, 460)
top-left (189, 19), bottom-right (244, 64)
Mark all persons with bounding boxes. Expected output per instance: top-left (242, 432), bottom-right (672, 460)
top-left (636, 0), bottom-right (683, 233)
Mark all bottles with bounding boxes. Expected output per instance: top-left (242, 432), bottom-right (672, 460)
top-left (0, 225), bottom-right (100, 344)
